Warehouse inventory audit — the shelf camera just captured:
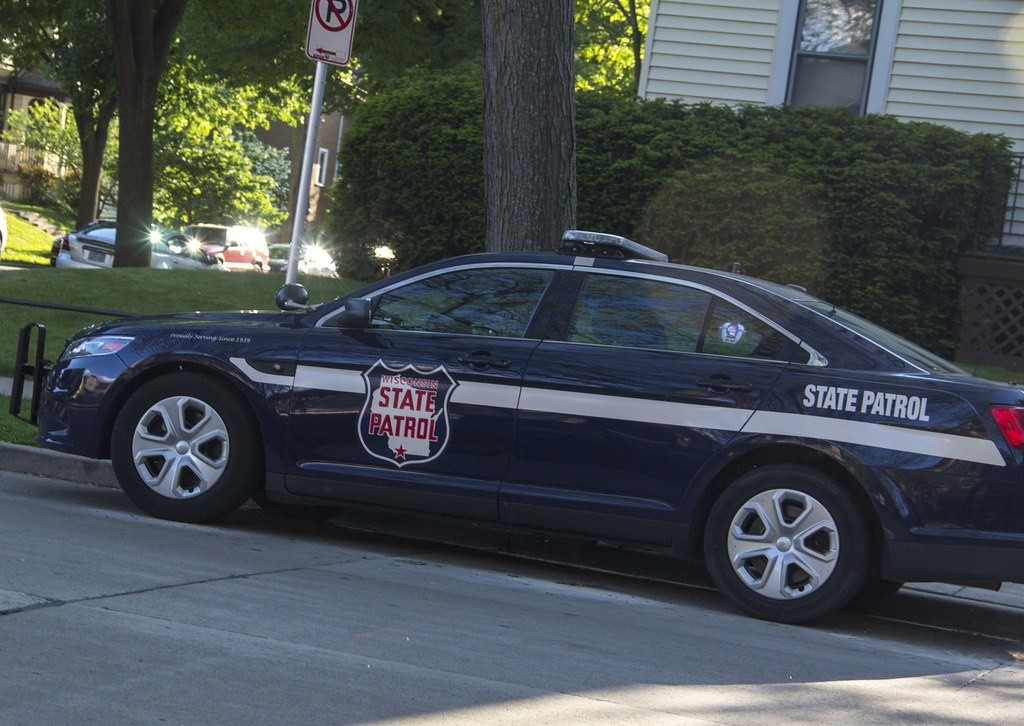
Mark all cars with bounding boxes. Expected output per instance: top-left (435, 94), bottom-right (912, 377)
top-left (48, 220), bottom-right (338, 277)
top-left (31, 226), bottom-right (1024, 627)
